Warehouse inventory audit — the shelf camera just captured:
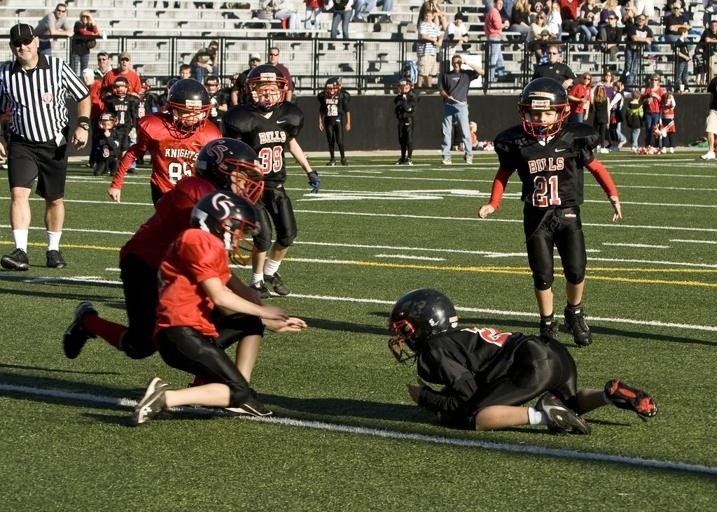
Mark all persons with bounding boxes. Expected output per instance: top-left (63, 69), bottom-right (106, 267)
top-left (478, 77), bottom-right (620, 346)
top-left (437, 54), bottom-right (494, 164)
top-left (33, 3), bottom-right (99, 77)
top-left (105, 65), bottom-right (320, 298)
top-left (394, 77), bottom-right (417, 165)
top-left (568, 72), bottom-right (676, 154)
top-left (257, 0), bottom-right (395, 40)
top-left (30, 41), bottom-right (351, 176)
top-left (414, 0), bottom-right (717, 94)
top-left (1, 24), bottom-right (91, 270)
top-left (63, 138), bottom-right (308, 423)
top-left (701, 76), bottom-right (717, 160)
top-left (385, 288), bottom-right (658, 433)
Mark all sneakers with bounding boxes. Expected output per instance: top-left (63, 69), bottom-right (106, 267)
top-left (248, 280), bottom-right (273, 300)
top-left (325, 158), bottom-right (351, 167)
top-left (603, 377), bottom-right (658, 418)
top-left (441, 156), bottom-right (454, 166)
top-left (700, 150), bottom-right (716, 161)
top-left (1, 247), bottom-right (30, 271)
top-left (594, 142), bottom-right (676, 156)
top-left (464, 155), bottom-right (475, 166)
top-left (539, 314), bottom-right (557, 341)
top-left (187, 381), bottom-right (277, 417)
top-left (132, 376), bottom-right (172, 426)
top-left (563, 301), bottom-right (594, 346)
top-left (264, 271), bottom-right (292, 296)
top-left (62, 302), bottom-right (98, 358)
top-left (46, 250), bottom-right (66, 268)
top-left (535, 390), bottom-right (594, 435)
top-left (393, 159), bottom-right (415, 166)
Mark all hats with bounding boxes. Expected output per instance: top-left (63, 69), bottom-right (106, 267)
top-left (96, 51), bottom-right (110, 60)
top-left (249, 52), bottom-right (261, 63)
top-left (8, 23), bottom-right (37, 49)
top-left (118, 52), bottom-right (132, 60)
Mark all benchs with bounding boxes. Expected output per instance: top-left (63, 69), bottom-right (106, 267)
top-left (0, 0), bottom-right (716, 97)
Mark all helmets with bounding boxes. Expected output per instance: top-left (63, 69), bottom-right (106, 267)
top-left (386, 286), bottom-right (462, 364)
top-left (517, 76), bottom-right (572, 138)
top-left (205, 74), bottom-right (221, 95)
top-left (98, 110), bottom-right (118, 129)
top-left (112, 76), bottom-right (130, 97)
top-left (244, 64), bottom-right (288, 112)
top-left (323, 78), bottom-right (341, 96)
top-left (165, 78), bottom-right (211, 135)
top-left (189, 191), bottom-right (263, 267)
top-left (397, 78), bottom-right (413, 95)
top-left (194, 138), bottom-right (266, 207)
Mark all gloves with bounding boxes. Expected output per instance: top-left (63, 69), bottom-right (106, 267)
top-left (306, 171), bottom-right (321, 194)
